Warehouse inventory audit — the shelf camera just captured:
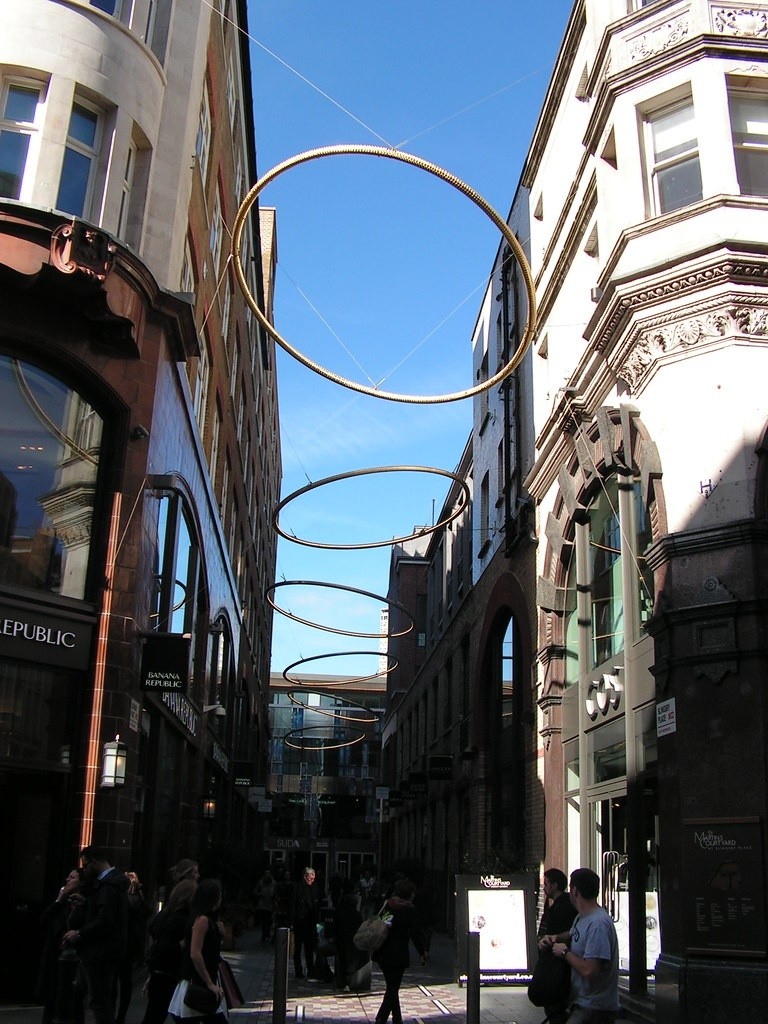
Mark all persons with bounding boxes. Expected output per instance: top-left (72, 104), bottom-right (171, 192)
top-left (254, 866), bottom-right (432, 1024)
top-left (40, 845), bottom-right (245, 1024)
top-left (537, 867), bottom-right (620, 1024)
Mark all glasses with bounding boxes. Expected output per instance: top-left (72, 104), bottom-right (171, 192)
top-left (83, 862), bottom-right (90, 869)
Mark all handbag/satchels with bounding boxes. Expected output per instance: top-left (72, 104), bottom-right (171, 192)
top-left (528, 948), bottom-right (572, 1006)
top-left (184, 977), bottom-right (221, 1013)
top-left (353, 900), bottom-right (394, 951)
top-left (217, 959), bottom-right (244, 1008)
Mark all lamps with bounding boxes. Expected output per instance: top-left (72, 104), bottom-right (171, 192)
top-left (98, 736), bottom-right (129, 792)
top-left (198, 793), bottom-right (218, 819)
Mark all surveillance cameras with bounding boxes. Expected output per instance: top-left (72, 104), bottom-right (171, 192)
top-left (214, 707), bottom-right (226, 719)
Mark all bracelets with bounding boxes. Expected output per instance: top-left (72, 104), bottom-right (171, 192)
top-left (560, 947), bottom-right (570, 958)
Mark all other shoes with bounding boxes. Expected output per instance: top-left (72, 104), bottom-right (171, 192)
top-left (307, 976), bottom-right (327, 983)
top-left (340, 984), bottom-right (351, 992)
top-left (295, 972), bottom-right (305, 978)
top-left (261, 933), bottom-right (270, 941)
